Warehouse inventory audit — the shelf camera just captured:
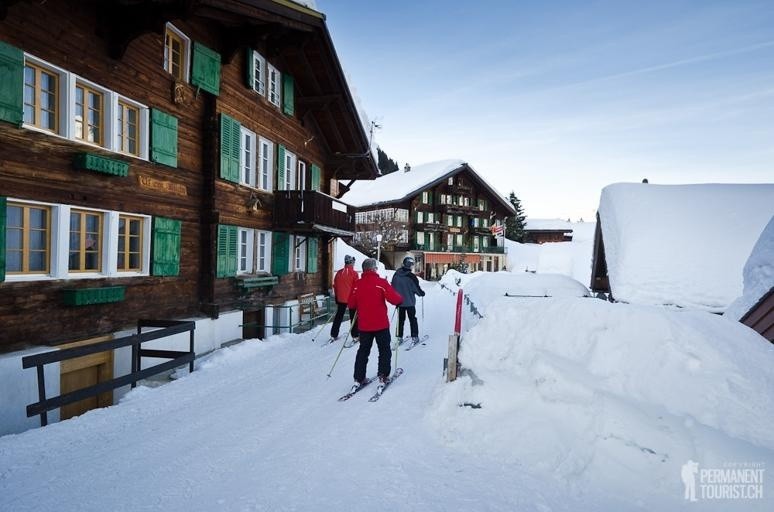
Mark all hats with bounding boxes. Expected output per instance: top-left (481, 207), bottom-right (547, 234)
top-left (345, 255), bottom-right (356, 265)
top-left (361, 259), bottom-right (379, 272)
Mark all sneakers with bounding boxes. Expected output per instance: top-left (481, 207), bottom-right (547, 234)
top-left (411, 337), bottom-right (419, 344)
top-left (352, 378), bottom-right (371, 388)
top-left (379, 374), bottom-right (391, 387)
top-left (395, 337), bottom-right (403, 345)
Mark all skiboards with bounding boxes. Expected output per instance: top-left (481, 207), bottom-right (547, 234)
top-left (389, 334), bottom-right (428, 352)
top-left (322, 330), bottom-right (359, 349)
top-left (336, 368), bottom-right (404, 403)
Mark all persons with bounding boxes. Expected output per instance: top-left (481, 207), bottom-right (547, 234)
top-left (391, 256), bottom-right (425, 342)
top-left (347, 257), bottom-right (404, 386)
top-left (331, 255), bottom-right (360, 342)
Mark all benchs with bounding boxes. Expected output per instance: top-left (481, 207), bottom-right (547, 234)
top-left (297, 288), bottom-right (328, 324)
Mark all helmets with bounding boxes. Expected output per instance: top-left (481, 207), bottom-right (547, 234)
top-left (402, 257), bottom-right (416, 268)
